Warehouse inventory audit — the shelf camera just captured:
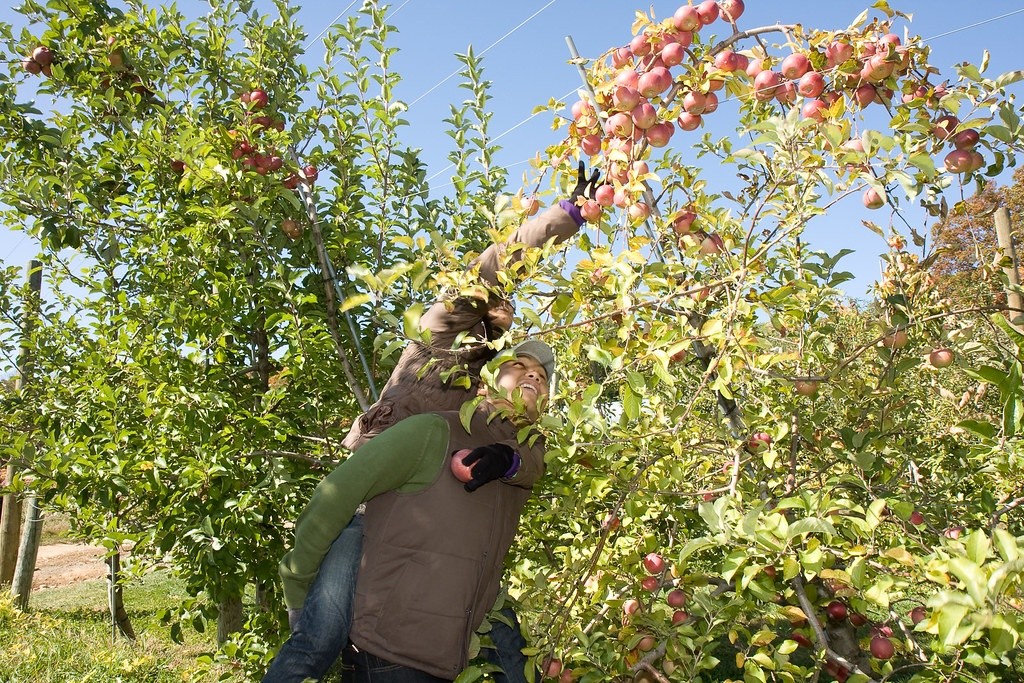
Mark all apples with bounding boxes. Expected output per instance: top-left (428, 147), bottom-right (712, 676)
top-left (171, 90), bottom-right (318, 238)
top-left (22, 46), bottom-right (53, 78)
top-left (518, 0), bottom-right (985, 683)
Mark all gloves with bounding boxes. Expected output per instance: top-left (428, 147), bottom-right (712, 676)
top-left (569, 160), bottom-right (614, 222)
top-left (451, 442), bottom-right (514, 493)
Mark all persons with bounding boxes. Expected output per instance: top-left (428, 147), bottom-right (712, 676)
top-left (280, 341), bottom-right (555, 683)
top-left (262, 159), bottom-right (611, 683)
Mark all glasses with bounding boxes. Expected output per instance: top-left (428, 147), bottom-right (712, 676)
top-left (507, 295), bottom-right (517, 314)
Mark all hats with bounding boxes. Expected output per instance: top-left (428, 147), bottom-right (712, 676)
top-left (494, 339), bottom-right (555, 387)
